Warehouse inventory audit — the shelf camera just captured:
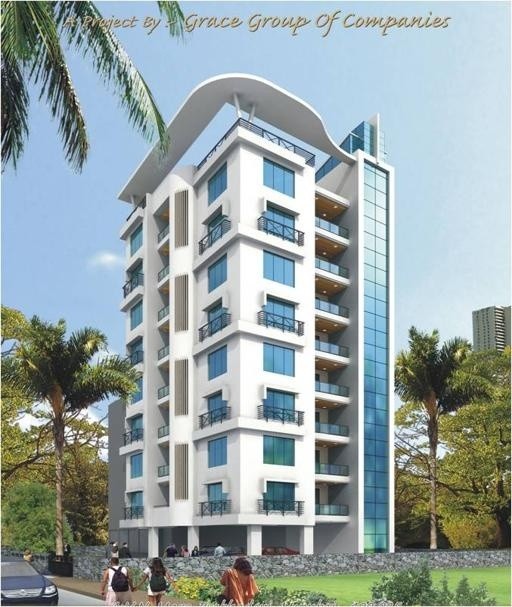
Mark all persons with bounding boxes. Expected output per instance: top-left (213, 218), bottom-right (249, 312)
top-left (132, 557), bottom-right (172, 605)
top-left (99, 557), bottom-right (135, 605)
top-left (23, 548), bottom-right (33, 562)
top-left (220, 557), bottom-right (260, 606)
top-left (109, 540), bottom-right (226, 559)
top-left (63, 539), bottom-right (71, 555)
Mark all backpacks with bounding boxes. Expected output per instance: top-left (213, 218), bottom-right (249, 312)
top-left (110, 566), bottom-right (129, 593)
top-left (149, 566), bottom-right (166, 592)
